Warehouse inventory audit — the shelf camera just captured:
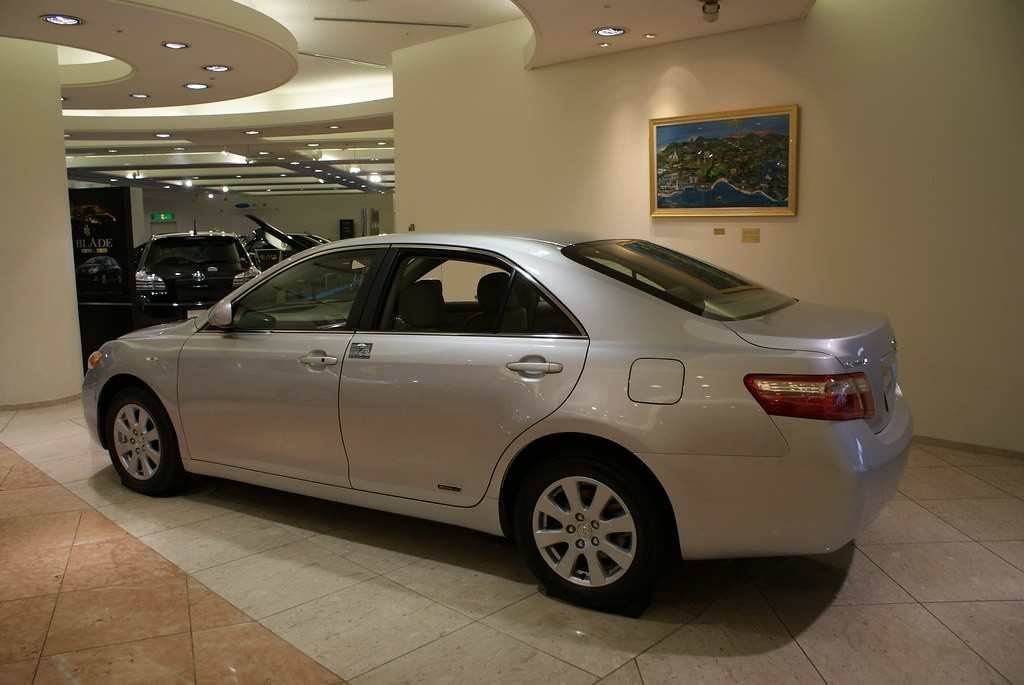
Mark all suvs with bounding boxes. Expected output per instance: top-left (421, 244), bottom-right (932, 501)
top-left (126, 230), bottom-right (267, 331)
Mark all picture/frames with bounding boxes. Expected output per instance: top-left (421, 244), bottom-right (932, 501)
top-left (648, 105), bottom-right (800, 218)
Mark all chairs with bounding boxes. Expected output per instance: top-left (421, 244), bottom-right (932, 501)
top-left (465, 272), bottom-right (544, 334)
top-left (394, 279), bottom-right (452, 334)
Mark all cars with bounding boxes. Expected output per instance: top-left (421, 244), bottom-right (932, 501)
top-left (70, 204), bottom-right (119, 240)
top-left (81, 229), bottom-right (915, 614)
top-left (236, 213), bottom-right (366, 301)
top-left (75, 256), bottom-right (124, 287)
